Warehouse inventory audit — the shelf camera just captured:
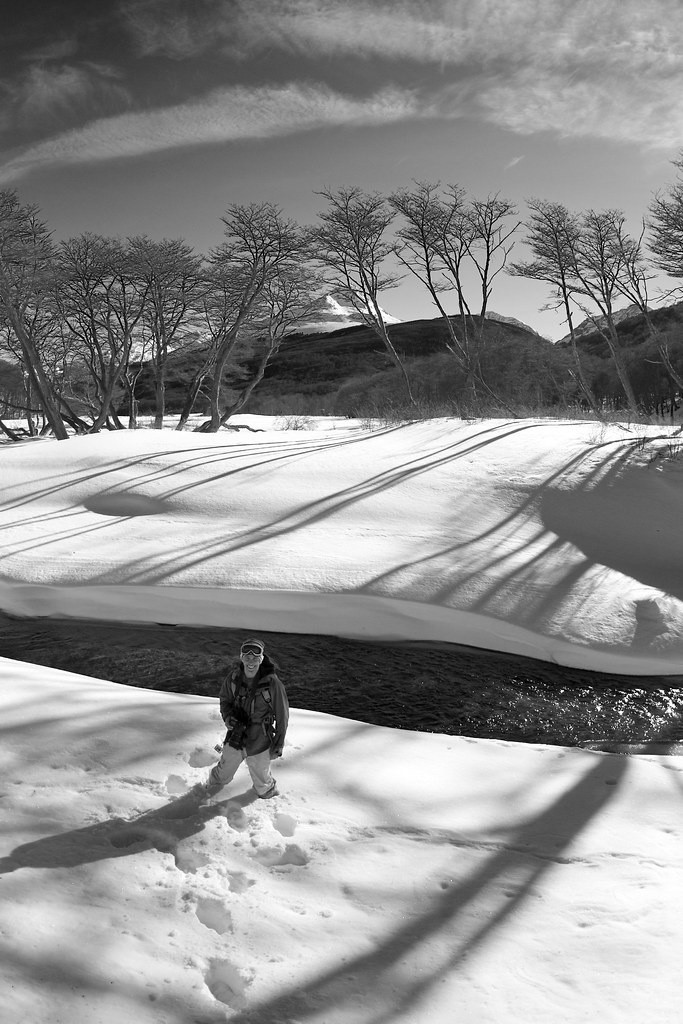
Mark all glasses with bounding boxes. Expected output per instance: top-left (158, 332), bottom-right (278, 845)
top-left (241, 644), bottom-right (263, 655)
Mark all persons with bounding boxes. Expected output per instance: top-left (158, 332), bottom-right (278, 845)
top-left (210, 638), bottom-right (289, 798)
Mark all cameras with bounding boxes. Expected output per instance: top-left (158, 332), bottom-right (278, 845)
top-left (228, 717), bottom-right (249, 751)
